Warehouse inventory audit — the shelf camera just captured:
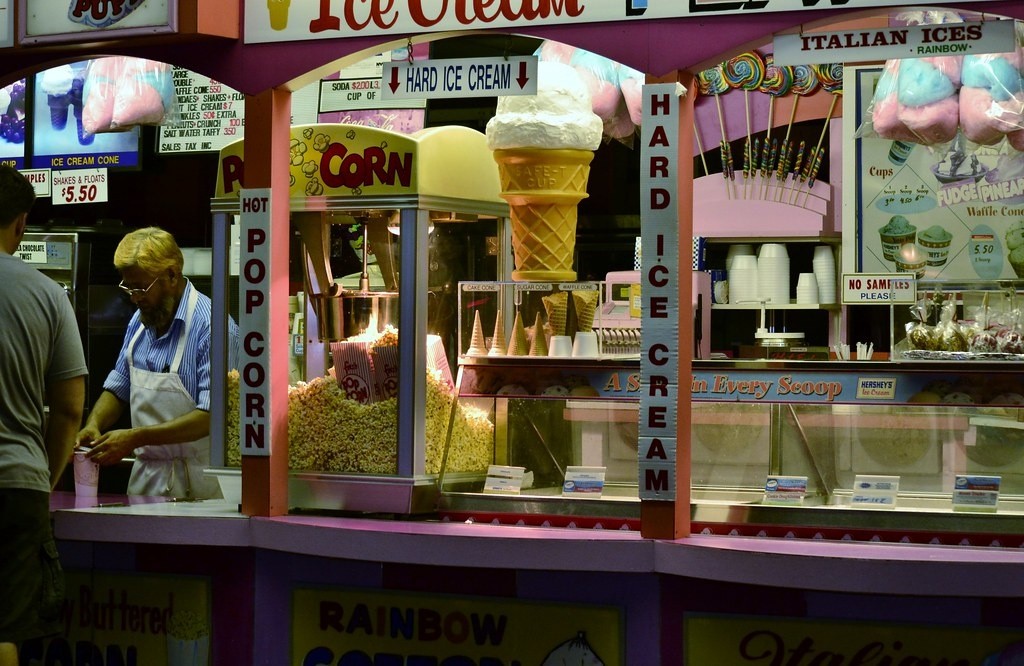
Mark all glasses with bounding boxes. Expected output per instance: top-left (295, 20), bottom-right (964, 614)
top-left (119, 277), bottom-right (160, 296)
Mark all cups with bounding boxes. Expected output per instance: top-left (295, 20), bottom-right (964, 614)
top-left (572, 332), bottom-right (598, 358)
top-left (893, 252), bottom-right (928, 280)
top-left (879, 225), bottom-right (916, 261)
top-left (888, 139), bottom-right (915, 167)
top-left (917, 231), bottom-right (953, 267)
top-left (267, 0), bottom-right (290, 31)
top-left (548, 336), bottom-right (572, 357)
top-left (72, 452), bottom-right (100, 508)
top-left (727, 244), bottom-right (837, 304)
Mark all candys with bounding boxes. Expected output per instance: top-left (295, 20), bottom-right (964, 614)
top-left (685, 50), bottom-right (843, 209)
top-left (905, 289), bottom-right (1024, 356)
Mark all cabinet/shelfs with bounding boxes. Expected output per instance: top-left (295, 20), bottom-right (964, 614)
top-left (693, 170), bottom-right (841, 362)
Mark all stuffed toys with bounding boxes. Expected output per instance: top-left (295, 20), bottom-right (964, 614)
top-left (871, 12), bottom-right (1024, 151)
top-left (81, 59), bottom-right (174, 135)
top-left (534, 41), bottom-right (644, 140)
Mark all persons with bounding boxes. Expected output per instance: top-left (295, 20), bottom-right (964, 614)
top-left (74, 227), bottom-right (239, 466)
top-left (0, 164), bottom-right (88, 666)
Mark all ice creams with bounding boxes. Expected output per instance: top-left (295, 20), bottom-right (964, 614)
top-left (931, 141), bottom-right (989, 184)
top-left (41, 64), bottom-right (74, 129)
top-left (893, 242), bottom-right (929, 278)
top-left (917, 225), bottom-right (953, 266)
top-left (1005, 220), bottom-right (1024, 278)
top-left (879, 214), bottom-right (918, 261)
top-left (485, 59), bottom-right (604, 282)
